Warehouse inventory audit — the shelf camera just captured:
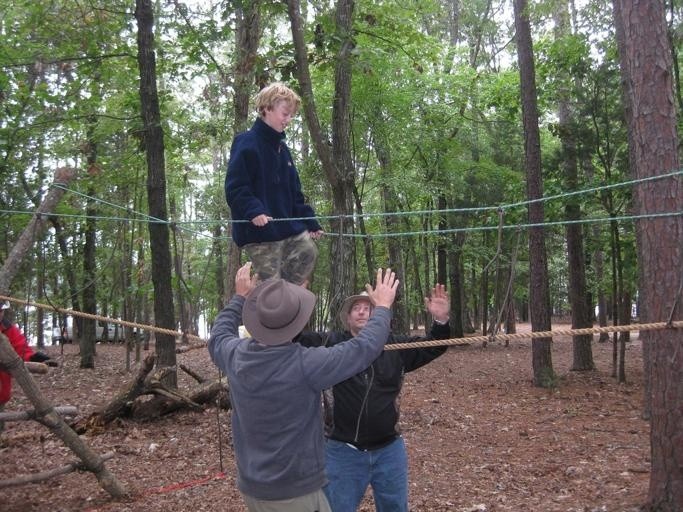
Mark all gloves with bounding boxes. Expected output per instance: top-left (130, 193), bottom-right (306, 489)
top-left (30, 351), bottom-right (50, 362)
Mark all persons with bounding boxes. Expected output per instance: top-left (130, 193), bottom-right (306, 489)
top-left (1, 300), bottom-right (50, 409)
top-left (225, 82), bottom-right (325, 285)
top-left (206, 261), bottom-right (400, 512)
top-left (293, 284), bottom-right (452, 512)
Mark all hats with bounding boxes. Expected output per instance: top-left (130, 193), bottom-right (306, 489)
top-left (339, 291), bottom-right (369, 332)
top-left (242, 278), bottom-right (316, 346)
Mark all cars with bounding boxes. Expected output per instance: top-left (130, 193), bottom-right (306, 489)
top-left (51, 307), bottom-right (146, 344)
